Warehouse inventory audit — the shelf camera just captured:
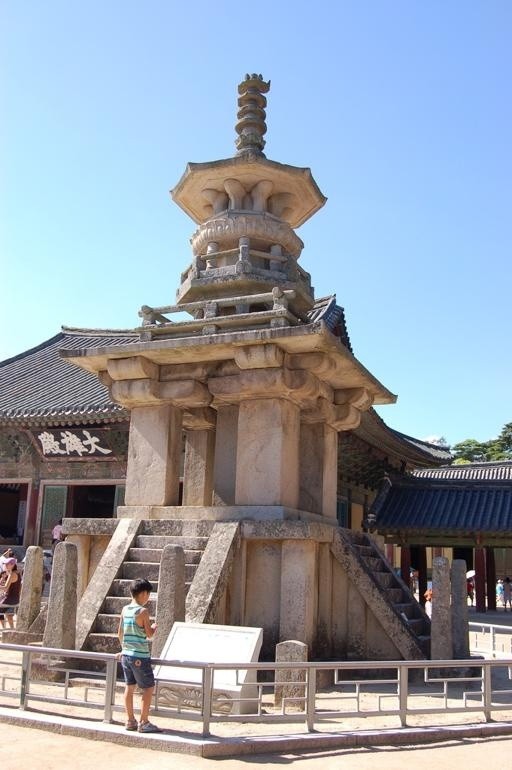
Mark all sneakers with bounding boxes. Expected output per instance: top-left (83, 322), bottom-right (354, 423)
top-left (137, 720), bottom-right (163, 732)
top-left (126, 718), bottom-right (138, 731)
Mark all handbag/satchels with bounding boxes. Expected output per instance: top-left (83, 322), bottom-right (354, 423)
top-left (45, 568), bottom-right (50, 582)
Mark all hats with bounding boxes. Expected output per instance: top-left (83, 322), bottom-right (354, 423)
top-left (2, 558), bottom-right (16, 565)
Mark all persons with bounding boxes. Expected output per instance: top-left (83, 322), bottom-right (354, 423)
top-left (0, 519), bottom-right (63, 628)
top-left (410, 568), bottom-right (511, 620)
top-left (123, 585), bottom-right (158, 732)
